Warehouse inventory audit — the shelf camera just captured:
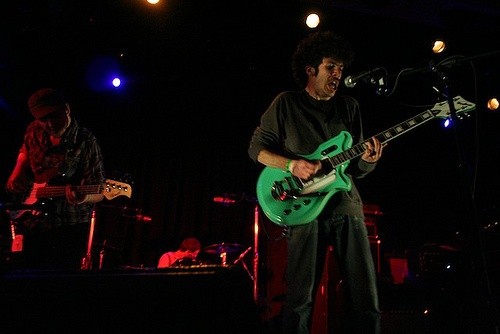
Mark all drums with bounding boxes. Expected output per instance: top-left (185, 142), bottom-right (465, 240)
top-left (176, 256), bottom-right (208, 266)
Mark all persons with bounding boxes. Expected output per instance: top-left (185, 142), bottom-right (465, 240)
top-left (247, 33), bottom-right (382, 334)
top-left (6, 88), bottom-right (104, 270)
top-left (158, 237), bottom-right (200, 268)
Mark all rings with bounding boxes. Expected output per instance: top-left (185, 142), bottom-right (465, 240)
top-left (372, 156), bottom-right (376, 159)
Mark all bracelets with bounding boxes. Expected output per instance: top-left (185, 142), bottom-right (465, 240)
top-left (286, 159), bottom-right (296, 173)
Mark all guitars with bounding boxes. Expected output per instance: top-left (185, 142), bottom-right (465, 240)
top-left (0, 172), bottom-right (137, 216)
top-left (255, 94), bottom-right (477, 228)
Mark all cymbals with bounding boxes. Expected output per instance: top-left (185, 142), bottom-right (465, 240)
top-left (205, 243), bottom-right (243, 255)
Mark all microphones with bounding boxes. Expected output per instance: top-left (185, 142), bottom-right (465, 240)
top-left (344, 68), bottom-right (379, 87)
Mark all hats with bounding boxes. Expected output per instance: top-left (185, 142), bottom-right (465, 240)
top-left (27, 89), bottom-right (65, 113)
